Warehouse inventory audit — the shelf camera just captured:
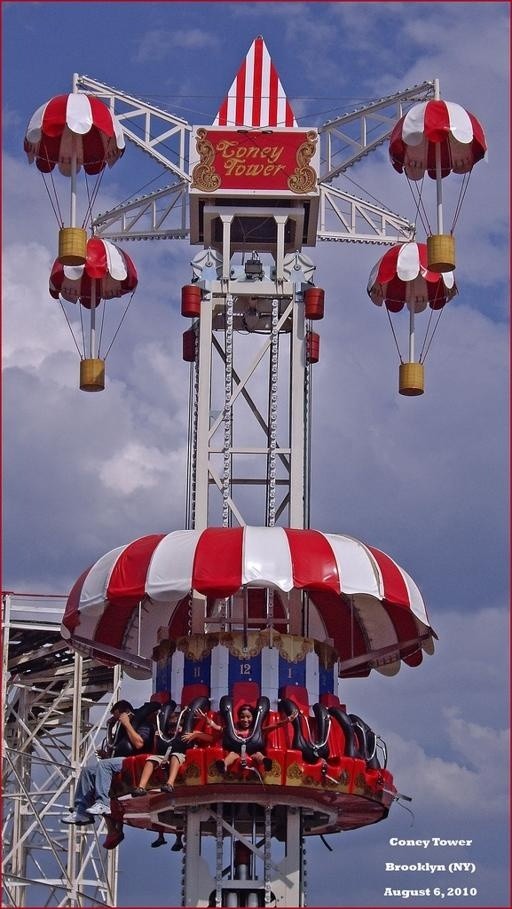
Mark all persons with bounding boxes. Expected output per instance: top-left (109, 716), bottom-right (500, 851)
top-left (59, 699), bottom-right (150, 823)
top-left (149, 832), bottom-right (184, 852)
top-left (101, 816), bottom-right (125, 849)
top-left (192, 702), bottom-right (301, 776)
top-left (130, 711), bottom-right (196, 799)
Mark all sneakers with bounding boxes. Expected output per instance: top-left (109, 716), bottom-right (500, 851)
top-left (160, 782), bottom-right (175, 793)
top-left (152, 837), bottom-right (165, 847)
top-left (61, 813), bottom-right (90, 824)
top-left (131, 786), bottom-right (146, 798)
top-left (216, 759), bottom-right (225, 773)
top-left (171, 841), bottom-right (183, 851)
top-left (103, 830), bottom-right (125, 850)
top-left (84, 803), bottom-right (112, 815)
top-left (263, 758), bottom-right (272, 771)
